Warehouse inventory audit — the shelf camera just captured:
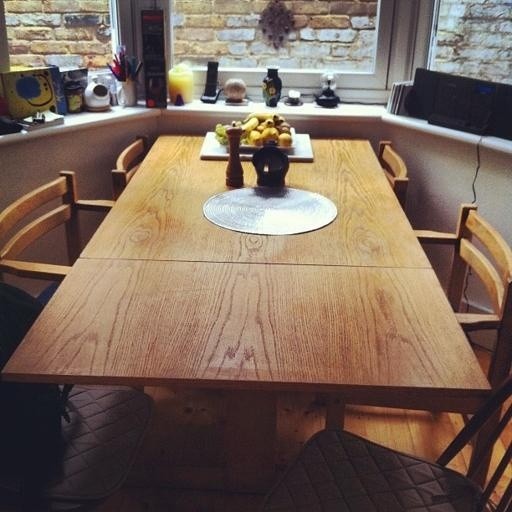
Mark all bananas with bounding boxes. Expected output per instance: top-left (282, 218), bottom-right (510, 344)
top-left (233, 112), bottom-right (285, 134)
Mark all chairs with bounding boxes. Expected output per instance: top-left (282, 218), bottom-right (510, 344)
top-left (258, 374), bottom-right (512, 509)
top-left (1, 283), bottom-right (154, 507)
top-left (112, 133), bottom-right (150, 196)
top-left (376, 138), bottom-right (412, 214)
top-left (324, 200), bottom-right (512, 492)
top-left (0, 168), bottom-right (117, 305)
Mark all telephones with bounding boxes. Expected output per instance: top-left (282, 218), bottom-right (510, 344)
top-left (200, 62), bottom-right (221, 103)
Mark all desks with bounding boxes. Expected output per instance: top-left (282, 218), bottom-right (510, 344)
top-left (3, 135), bottom-right (496, 498)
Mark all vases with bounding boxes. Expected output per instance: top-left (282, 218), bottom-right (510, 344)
top-left (263, 69), bottom-right (281, 106)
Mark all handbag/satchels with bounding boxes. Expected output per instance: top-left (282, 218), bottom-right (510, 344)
top-left (1, 282), bottom-right (62, 450)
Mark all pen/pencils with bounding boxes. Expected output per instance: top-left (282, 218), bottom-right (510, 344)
top-left (107, 47), bottom-right (143, 81)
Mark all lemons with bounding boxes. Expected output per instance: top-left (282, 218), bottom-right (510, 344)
top-left (246, 127), bottom-right (292, 147)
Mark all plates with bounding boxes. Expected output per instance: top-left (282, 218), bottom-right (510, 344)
top-left (226, 127), bottom-right (296, 152)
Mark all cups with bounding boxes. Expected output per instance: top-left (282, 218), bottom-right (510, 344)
top-left (114, 79), bottom-right (137, 107)
